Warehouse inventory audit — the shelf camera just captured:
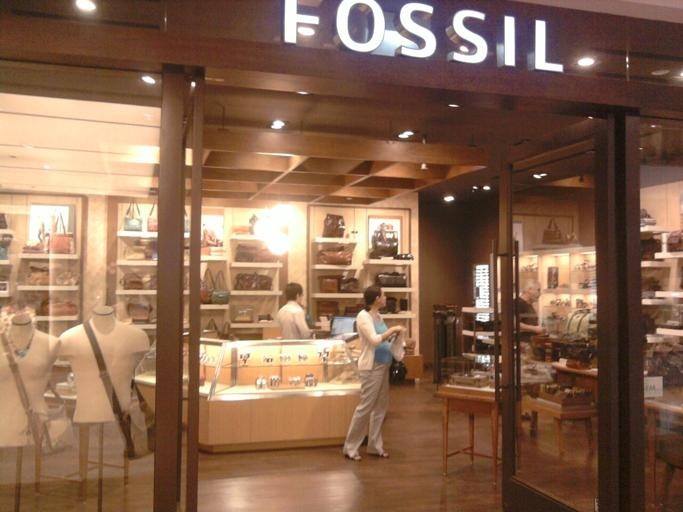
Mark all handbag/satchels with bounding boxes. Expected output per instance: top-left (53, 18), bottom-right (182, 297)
top-left (116, 401), bottom-right (154, 460)
top-left (316, 243), bottom-right (357, 265)
top-left (234, 243), bottom-right (277, 262)
top-left (122, 271), bottom-right (144, 290)
top-left (638, 231), bottom-right (683, 262)
top-left (389, 359), bottom-right (408, 387)
top-left (372, 236), bottom-right (398, 257)
top-left (315, 299), bottom-right (364, 321)
top-left (146, 216), bottom-right (158, 232)
top-left (129, 303), bottom-right (158, 323)
top-left (24, 265), bottom-right (80, 286)
top-left (122, 215), bottom-right (143, 231)
top-left (377, 297), bottom-right (400, 315)
top-left (123, 245), bottom-right (145, 261)
top-left (373, 270), bottom-right (407, 288)
top-left (318, 275), bottom-right (360, 292)
top-left (234, 273), bottom-right (273, 290)
top-left (230, 304), bottom-right (254, 323)
top-left (49, 233), bottom-right (74, 254)
top-left (200, 329), bottom-right (235, 342)
top-left (540, 228), bottom-right (563, 244)
top-left (26, 403), bottom-right (75, 456)
top-left (198, 267), bottom-right (229, 304)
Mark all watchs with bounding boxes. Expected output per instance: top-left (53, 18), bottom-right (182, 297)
top-left (257, 373), bottom-right (318, 387)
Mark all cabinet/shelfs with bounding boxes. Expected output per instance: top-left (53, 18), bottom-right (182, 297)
top-left (311, 237), bottom-right (415, 320)
top-left (532, 225), bottom-right (683, 344)
top-left (0, 230), bottom-right (79, 368)
top-left (461, 306), bottom-right (502, 363)
top-left (133, 336), bottom-right (368, 454)
top-left (115, 230), bottom-right (282, 331)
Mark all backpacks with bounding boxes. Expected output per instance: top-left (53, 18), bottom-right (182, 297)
top-left (40, 297), bottom-right (78, 316)
top-left (321, 213), bottom-right (345, 238)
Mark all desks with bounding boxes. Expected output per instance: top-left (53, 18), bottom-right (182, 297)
top-left (34, 382), bottom-right (128, 503)
top-left (433, 380), bottom-right (539, 488)
top-left (525, 394), bottom-right (596, 455)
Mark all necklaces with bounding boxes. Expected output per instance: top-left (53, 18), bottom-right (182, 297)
top-left (9, 328), bottom-right (34, 358)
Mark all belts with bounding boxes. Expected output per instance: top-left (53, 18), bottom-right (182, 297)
top-left (430, 304), bottom-right (463, 386)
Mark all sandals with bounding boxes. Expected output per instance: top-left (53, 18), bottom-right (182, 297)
top-left (343, 453), bottom-right (361, 461)
top-left (367, 450), bottom-right (388, 457)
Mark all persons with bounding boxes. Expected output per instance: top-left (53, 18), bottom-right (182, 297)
top-left (513, 279), bottom-right (547, 343)
top-left (0, 313), bottom-right (61, 448)
top-left (343, 287), bottom-right (405, 462)
top-left (278, 283), bottom-right (314, 340)
top-left (58, 304), bottom-right (151, 425)
